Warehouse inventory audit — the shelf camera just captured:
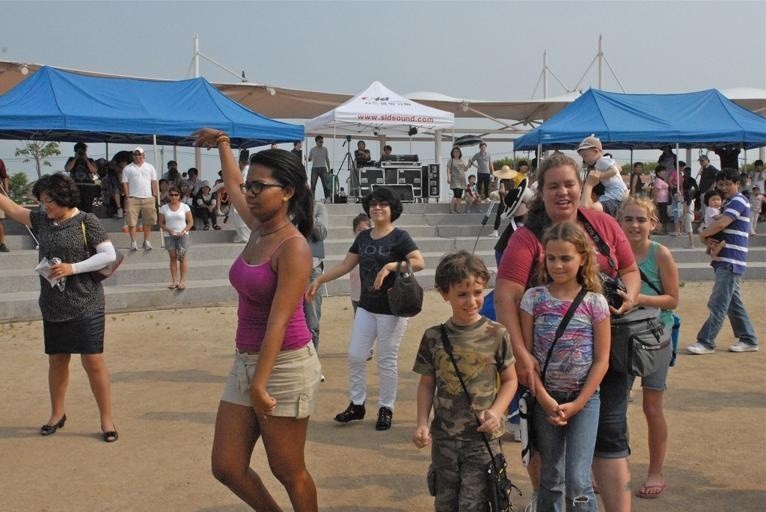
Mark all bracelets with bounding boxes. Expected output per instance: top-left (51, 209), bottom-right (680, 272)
top-left (214, 131), bottom-right (231, 146)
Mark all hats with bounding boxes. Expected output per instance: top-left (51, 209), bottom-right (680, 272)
top-left (576, 137), bottom-right (603, 152)
top-left (177, 179), bottom-right (212, 190)
top-left (500, 177), bottom-right (529, 221)
top-left (492, 165), bottom-right (518, 179)
top-left (133, 146), bottom-right (145, 154)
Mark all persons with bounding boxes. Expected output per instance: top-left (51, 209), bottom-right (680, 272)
top-left (516, 220), bottom-right (612, 511)
top-left (303, 189), bottom-right (426, 431)
top-left (347, 214), bottom-right (376, 363)
top-left (686, 166), bottom-right (761, 356)
top-left (0, 171), bottom-right (119, 445)
top-left (184, 128), bottom-right (324, 511)
top-left (615, 192), bottom-right (681, 501)
top-left (410, 248), bottom-right (520, 511)
top-left (495, 148), bottom-right (643, 511)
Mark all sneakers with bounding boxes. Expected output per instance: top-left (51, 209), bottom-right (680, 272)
top-left (376, 407), bottom-right (393, 431)
top-left (656, 228), bottom-right (695, 249)
top-left (727, 341), bottom-right (759, 352)
top-left (142, 239), bottom-right (152, 250)
top-left (685, 341), bottom-right (715, 354)
top-left (130, 240), bottom-right (138, 251)
top-left (488, 229), bottom-right (499, 238)
top-left (335, 400), bottom-right (366, 422)
top-left (524, 495), bottom-right (539, 512)
top-left (215, 208), bottom-right (226, 216)
top-left (0, 242), bottom-right (11, 253)
top-left (495, 429), bottom-right (515, 443)
top-left (448, 208), bottom-right (485, 215)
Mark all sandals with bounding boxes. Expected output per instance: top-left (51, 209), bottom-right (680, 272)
top-left (201, 224), bottom-right (222, 231)
top-left (167, 283), bottom-right (178, 289)
top-left (178, 282), bottom-right (186, 290)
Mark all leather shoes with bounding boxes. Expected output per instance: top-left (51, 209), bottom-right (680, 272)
top-left (42, 413), bottom-right (66, 435)
top-left (100, 422), bottom-right (121, 441)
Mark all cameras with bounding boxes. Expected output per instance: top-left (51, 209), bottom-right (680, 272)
top-left (598, 272), bottom-right (626, 319)
top-left (79, 150), bottom-right (84, 156)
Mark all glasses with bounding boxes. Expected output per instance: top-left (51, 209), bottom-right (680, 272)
top-left (239, 181), bottom-right (285, 195)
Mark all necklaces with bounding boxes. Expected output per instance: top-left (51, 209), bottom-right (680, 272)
top-left (253, 220), bottom-right (294, 246)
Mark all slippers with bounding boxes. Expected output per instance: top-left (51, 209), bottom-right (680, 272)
top-left (634, 479), bottom-right (668, 499)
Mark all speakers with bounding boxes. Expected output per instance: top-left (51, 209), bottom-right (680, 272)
top-left (371, 184), bottom-right (415, 203)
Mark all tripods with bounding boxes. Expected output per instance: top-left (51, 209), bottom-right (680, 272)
top-left (324, 135), bottom-right (364, 204)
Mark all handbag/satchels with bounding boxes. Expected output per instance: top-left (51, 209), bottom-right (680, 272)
top-left (87, 245), bottom-right (124, 281)
top-left (387, 274), bottom-right (423, 318)
top-left (605, 307), bottom-right (674, 379)
top-left (484, 451), bottom-right (513, 512)
top-left (517, 389), bottom-right (536, 466)
